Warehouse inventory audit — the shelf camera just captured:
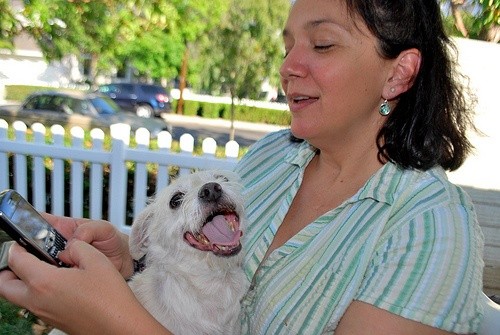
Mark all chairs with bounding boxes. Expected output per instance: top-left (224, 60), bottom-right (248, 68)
top-left (479, 290), bottom-right (500, 335)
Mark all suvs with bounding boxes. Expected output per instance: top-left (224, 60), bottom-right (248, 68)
top-left (93, 83), bottom-right (172, 119)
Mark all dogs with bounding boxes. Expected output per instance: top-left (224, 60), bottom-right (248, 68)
top-left (47, 166), bottom-right (247, 335)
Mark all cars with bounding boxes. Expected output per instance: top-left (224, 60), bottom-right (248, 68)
top-left (0, 92), bottom-right (168, 142)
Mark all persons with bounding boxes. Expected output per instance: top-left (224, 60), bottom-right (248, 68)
top-left (1, 1), bottom-right (484, 334)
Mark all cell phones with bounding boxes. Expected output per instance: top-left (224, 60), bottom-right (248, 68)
top-left (0, 189), bottom-right (73, 270)
